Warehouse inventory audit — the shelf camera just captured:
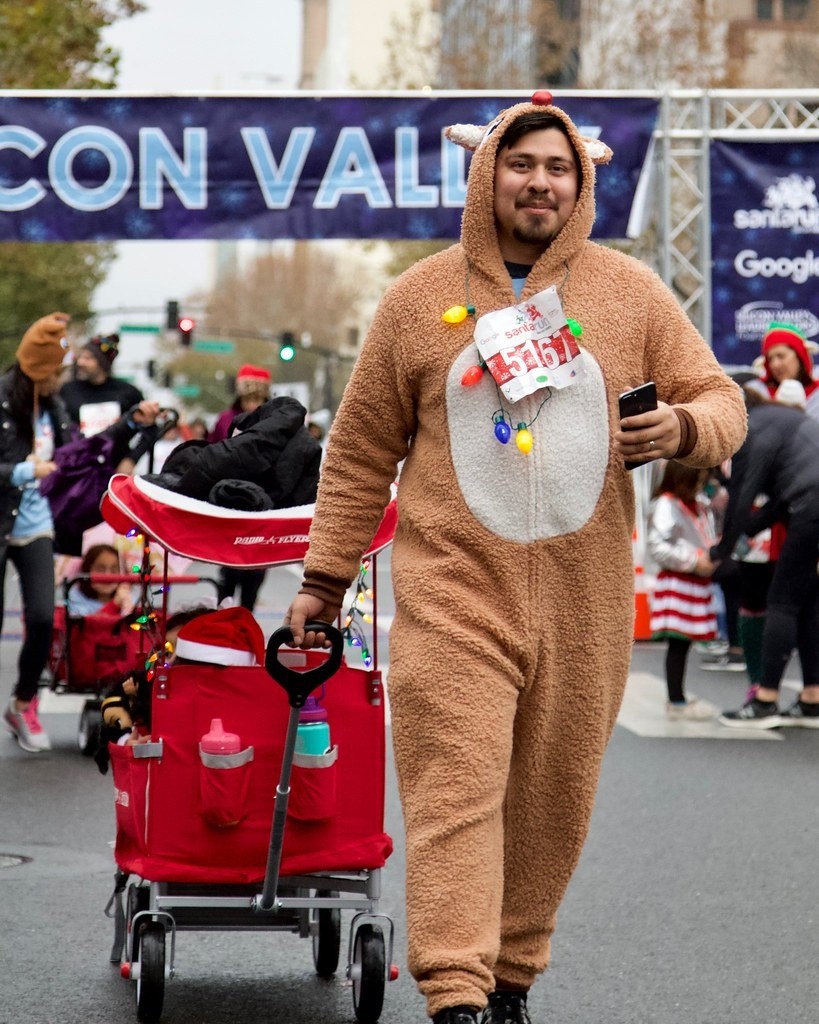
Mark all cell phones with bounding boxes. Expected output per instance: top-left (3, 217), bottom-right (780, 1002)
top-left (618, 381), bottom-right (658, 469)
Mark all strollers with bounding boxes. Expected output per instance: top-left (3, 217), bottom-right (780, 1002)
top-left (98, 472), bottom-right (399, 1024)
top-left (50, 572), bottom-right (226, 754)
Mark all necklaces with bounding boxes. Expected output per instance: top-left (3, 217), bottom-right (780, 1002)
top-left (441, 255), bottom-right (584, 457)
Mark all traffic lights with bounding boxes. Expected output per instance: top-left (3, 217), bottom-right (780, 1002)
top-left (179, 319), bottom-right (193, 346)
top-left (280, 332), bottom-right (296, 361)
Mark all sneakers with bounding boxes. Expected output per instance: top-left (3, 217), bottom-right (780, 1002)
top-left (780, 693), bottom-right (819, 727)
top-left (481, 989), bottom-right (531, 1024)
top-left (717, 683), bottom-right (783, 729)
top-left (3, 696), bottom-right (51, 753)
top-left (668, 699), bottom-right (716, 720)
top-left (432, 1006), bottom-right (477, 1024)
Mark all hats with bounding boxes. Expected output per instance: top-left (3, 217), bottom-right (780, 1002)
top-left (235, 365), bottom-right (270, 397)
top-left (16, 312), bottom-right (75, 420)
top-left (84, 335), bottom-right (120, 371)
top-left (761, 320), bottom-right (812, 382)
top-left (175, 606), bottom-right (265, 666)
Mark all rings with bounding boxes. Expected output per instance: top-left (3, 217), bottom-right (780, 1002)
top-left (649, 441), bottom-right (654, 451)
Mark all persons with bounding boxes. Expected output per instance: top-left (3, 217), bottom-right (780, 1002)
top-left (308, 408), bottom-right (334, 448)
top-left (117, 604), bottom-right (219, 747)
top-left (173, 604), bottom-right (269, 668)
top-left (132, 416), bottom-right (210, 477)
top-left (648, 459), bottom-right (723, 721)
top-left (283, 90), bottom-right (751, 1024)
top-left (708, 385), bottom-right (819, 730)
top-left (694, 319), bottom-right (819, 673)
top-left (0, 311), bottom-right (160, 752)
top-left (66, 544), bottom-right (140, 617)
top-left (98, 670), bottom-right (154, 736)
top-left (205, 362), bottom-right (271, 612)
top-left (61, 333), bottom-right (145, 441)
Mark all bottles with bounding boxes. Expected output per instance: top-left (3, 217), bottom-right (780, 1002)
top-left (294, 698), bottom-right (331, 757)
top-left (201, 719), bottom-right (241, 756)
